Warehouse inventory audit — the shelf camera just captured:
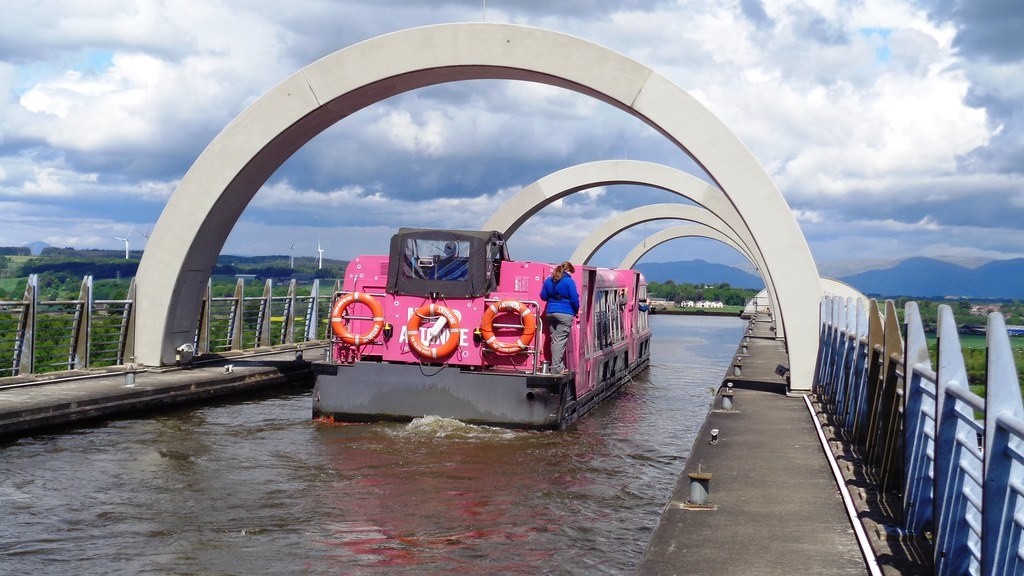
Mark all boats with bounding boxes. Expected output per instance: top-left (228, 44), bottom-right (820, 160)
top-left (309, 227), bottom-right (655, 434)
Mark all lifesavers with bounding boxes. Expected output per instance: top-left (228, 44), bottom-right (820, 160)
top-left (332, 293), bottom-right (534, 358)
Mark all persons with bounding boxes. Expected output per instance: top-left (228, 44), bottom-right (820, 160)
top-left (431, 241), bottom-right (468, 281)
top-left (620, 287), bottom-right (651, 312)
top-left (540, 261), bottom-right (580, 374)
